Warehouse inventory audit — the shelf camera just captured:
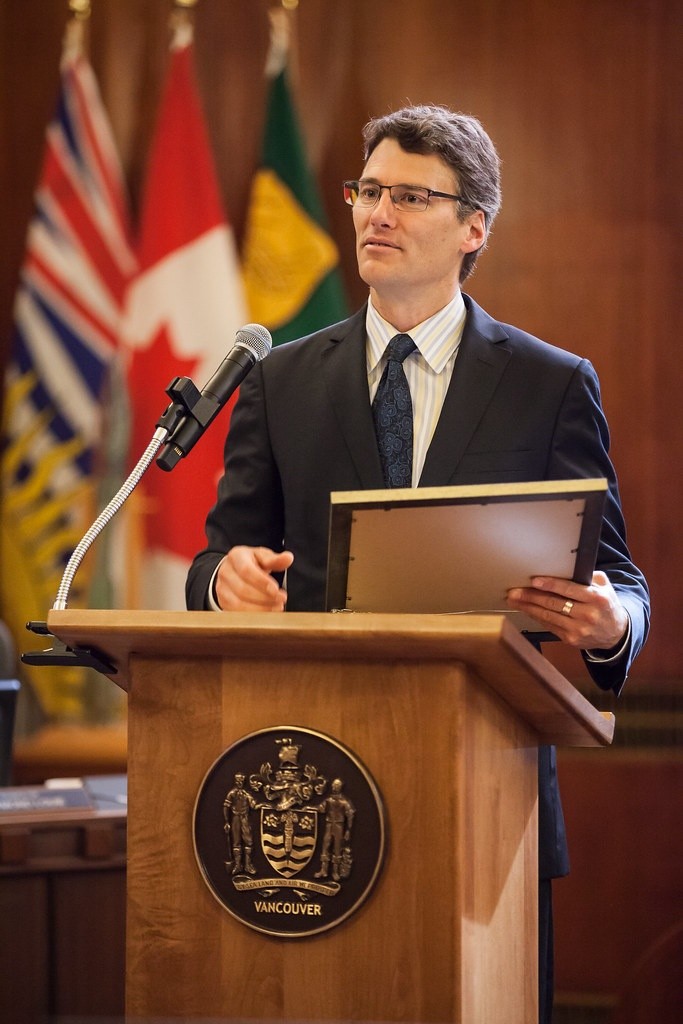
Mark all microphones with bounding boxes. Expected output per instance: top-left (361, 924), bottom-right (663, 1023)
top-left (156, 323), bottom-right (272, 471)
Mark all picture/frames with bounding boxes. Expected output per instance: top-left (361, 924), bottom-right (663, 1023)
top-left (324, 479), bottom-right (606, 641)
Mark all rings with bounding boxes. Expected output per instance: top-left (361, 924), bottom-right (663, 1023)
top-left (562, 601), bottom-right (574, 616)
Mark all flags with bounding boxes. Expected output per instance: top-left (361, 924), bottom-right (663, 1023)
top-left (240, 71), bottom-right (352, 347)
top-left (99, 30), bottom-right (246, 610)
top-left (0, 46), bottom-right (134, 722)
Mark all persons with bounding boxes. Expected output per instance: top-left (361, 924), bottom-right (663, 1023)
top-left (183, 106), bottom-right (654, 1024)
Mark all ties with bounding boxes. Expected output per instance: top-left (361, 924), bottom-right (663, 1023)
top-left (370, 333), bottom-right (418, 490)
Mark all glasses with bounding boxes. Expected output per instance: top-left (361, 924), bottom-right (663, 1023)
top-left (342, 180), bottom-right (468, 212)
